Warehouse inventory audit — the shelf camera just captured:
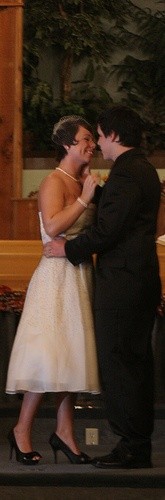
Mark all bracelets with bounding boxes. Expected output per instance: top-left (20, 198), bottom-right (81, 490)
top-left (77, 198), bottom-right (88, 208)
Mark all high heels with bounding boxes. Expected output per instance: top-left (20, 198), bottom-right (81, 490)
top-left (49, 432), bottom-right (92, 464)
top-left (10, 426), bottom-right (42, 464)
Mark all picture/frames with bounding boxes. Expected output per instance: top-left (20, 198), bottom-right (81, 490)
top-left (12, 0), bottom-right (165, 201)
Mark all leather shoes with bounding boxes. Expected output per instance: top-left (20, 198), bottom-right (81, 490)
top-left (93, 448), bottom-right (154, 469)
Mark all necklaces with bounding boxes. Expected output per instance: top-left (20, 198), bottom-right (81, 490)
top-left (56, 167), bottom-right (78, 182)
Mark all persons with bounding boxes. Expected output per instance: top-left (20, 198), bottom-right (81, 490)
top-left (42, 107), bottom-right (161, 469)
top-left (4, 115), bottom-right (110, 466)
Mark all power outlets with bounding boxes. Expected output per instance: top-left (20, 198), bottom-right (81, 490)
top-left (85, 428), bottom-right (98, 446)
